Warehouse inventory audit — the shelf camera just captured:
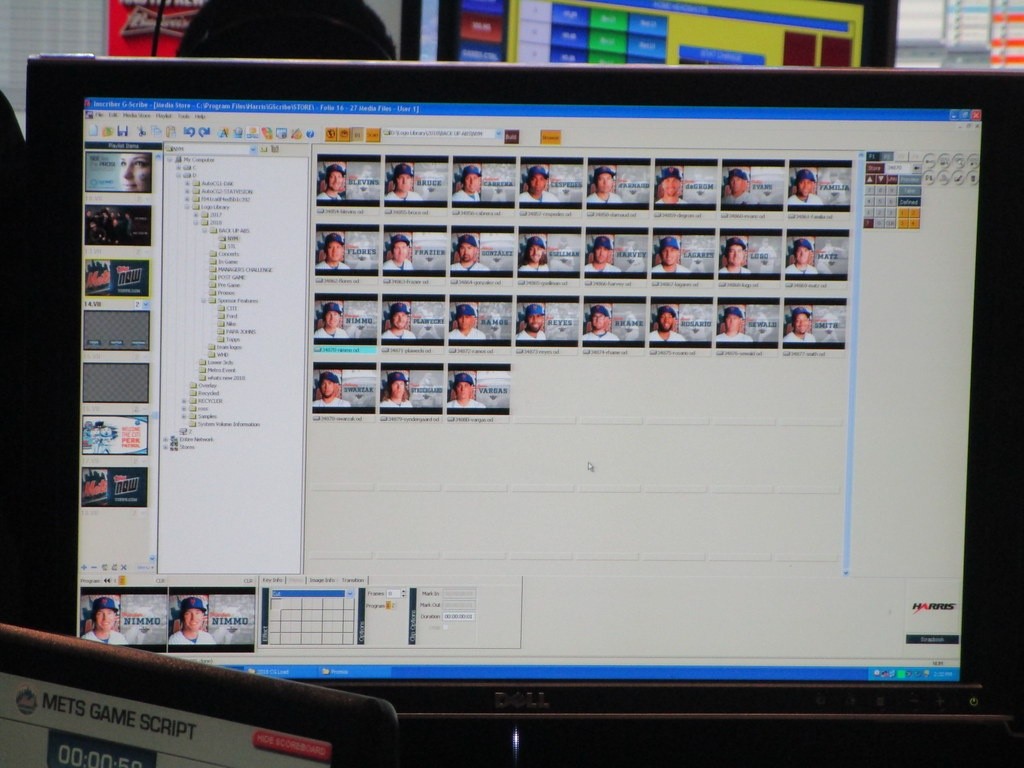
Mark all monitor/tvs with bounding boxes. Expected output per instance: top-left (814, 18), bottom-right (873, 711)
top-left (10, 54), bottom-right (1023, 768)
top-left (400, 0), bottom-right (900, 69)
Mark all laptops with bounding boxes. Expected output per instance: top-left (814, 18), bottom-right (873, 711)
top-left (0, 624), bottom-right (398, 768)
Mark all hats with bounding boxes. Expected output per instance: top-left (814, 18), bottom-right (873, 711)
top-left (322, 163), bottom-right (817, 318)
top-left (454, 373), bottom-right (474, 385)
top-left (178, 596), bottom-right (206, 619)
top-left (318, 371), bottom-right (340, 384)
top-left (387, 371), bottom-right (409, 386)
top-left (91, 597), bottom-right (120, 617)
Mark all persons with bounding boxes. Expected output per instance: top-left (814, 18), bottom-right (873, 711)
top-left (317, 161), bottom-right (821, 408)
top-left (119, 154), bottom-right (151, 192)
top-left (86, 206), bottom-right (133, 244)
top-left (80, 597), bottom-right (129, 645)
top-left (169, 597), bottom-right (216, 644)
